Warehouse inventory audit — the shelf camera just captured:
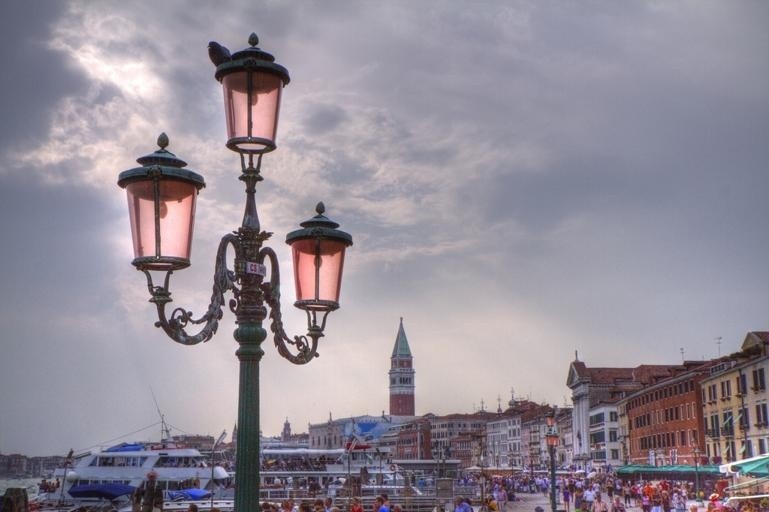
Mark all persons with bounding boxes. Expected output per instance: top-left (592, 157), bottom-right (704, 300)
top-left (131, 453), bottom-right (768, 511)
top-left (38, 475), bottom-right (61, 493)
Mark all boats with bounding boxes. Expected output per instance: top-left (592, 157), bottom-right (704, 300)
top-left (24, 419), bottom-right (426, 500)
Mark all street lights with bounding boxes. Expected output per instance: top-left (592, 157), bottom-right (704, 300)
top-left (473, 428), bottom-right (490, 512)
top-left (689, 438), bottom-right (703, 500)
top-left (541, 412), bottom-right (562, 512)
top-left (528, 448), bottom-right (535, 477)
top-left (117, 31), bottom-right (352, 512)
